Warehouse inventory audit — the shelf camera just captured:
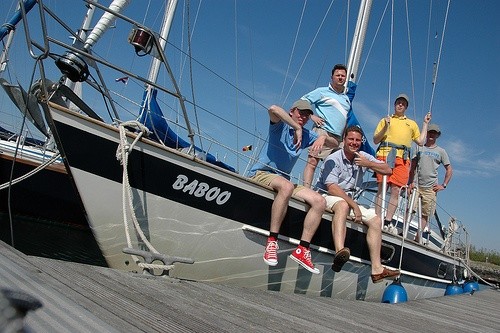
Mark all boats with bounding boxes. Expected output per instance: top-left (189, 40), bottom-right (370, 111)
top-left (1, 1), bottom-right (98, 177)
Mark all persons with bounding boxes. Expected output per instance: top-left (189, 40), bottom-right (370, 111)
top-left (311, 125), bottom-right (400, 283)
top-left (247, 100), bottom-right (338, 275)
top-left (374, 95), bottom-right (432, 235)
top-left (300, 64), bottom-right (354, 198)
top-left (400, 124), bottom-right (453, 247)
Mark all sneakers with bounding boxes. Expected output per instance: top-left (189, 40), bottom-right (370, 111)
top-left (415, 236), bottom-right (427, 245)
top-left (290, 244), bottom-right (320, 275)
top-left (263, 237), bottom-right (279, 266)
top-left (371, 267), bottom-right (400, 283)
top-left (331, 247), bottom-right (350, 273)
top-left (383, 223), bottom-right (397, 236)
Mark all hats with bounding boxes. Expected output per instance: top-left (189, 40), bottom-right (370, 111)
top-left (290, 100), bottom-right (313, 115)
top-left (427, 123), bottom-right (441, 138)
top-left (394, 94), bottom-right (409, 108)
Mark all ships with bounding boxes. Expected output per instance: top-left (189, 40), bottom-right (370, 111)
top-left (1, 0), bottom-right (471, 300)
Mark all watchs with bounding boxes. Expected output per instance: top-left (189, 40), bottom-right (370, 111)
top-left (442, 183), bottom-right (446, 189)
